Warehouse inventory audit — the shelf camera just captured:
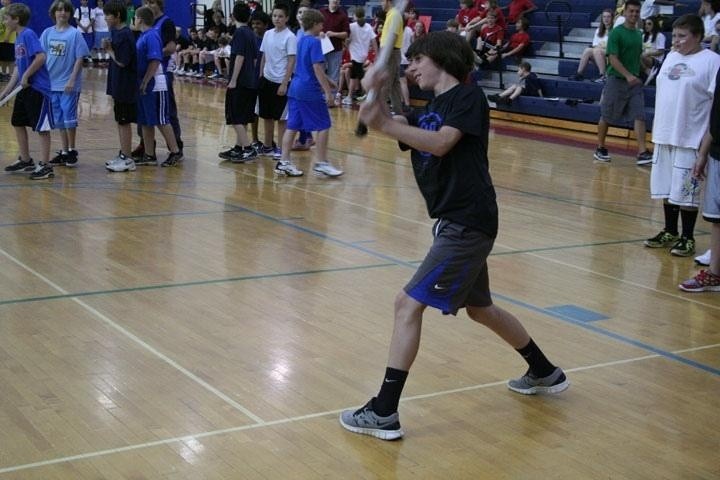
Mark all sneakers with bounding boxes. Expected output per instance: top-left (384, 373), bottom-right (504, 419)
top-left (314, 160), bottom-right (344, 176)
top-left (497, 98), bottom-right (512, 105)
top-left (472, 50), bottom-right (488, 66)
top-left (273, 147), bottom-right (282, 159)
top-left (250, 141), bottom-right (262, 150)
top-left (594, 75), bottom-right (607, 82)
top-left (636, 148), bottom-right (653, 165)
top-left (105, 154), bottom-right (136, 171)
top-left (218, 148), bottom-right (240, 158)
top-left (161, 150), bottom-right (185, 167)
top-left (274, 161), bottom-right (304, 176)
top-left (257, 143), bottom-right (273, 156)
top-left (177, 66), bottom-right (185, 75)
top-left (207, 73), bottom-right (218, 78)
top-left (5, 155), bottom-right (36, 172)
top-left (307, 136), bottom-right (316, 146)
top-left (292, 140), bottom-right (309, 150)
top-left (356, 95), bottom-right (366, 101)
top-left (487, 93), bottom-right (499, 101)
top-left (212, 74), bottom-right (224, 79)
top-left (231, 148), bottom-right (257, 162)
top-left (506, 366), bottom-right (569, 395)
top-left (48, 150), bottom-right (67, 165)
top-left (186, 69), bottom-right (197, 76)
top-left (670, 235), bottom-right (696, 256)
top-left (593, 147), bottom-right (611, 162)
top-left (333, 97), bottom-right (340, 106)
top-left (134, 154), bottom-right (158, 165)
top-left (105, 150), bottom-right (122, 165)
top-left (65, 150), bottom-right (78, 166)
top-left (338, 397), bottom-right (403, 441)
top-left (694, 248), bottom-right (712, 265)
top-left (28, 161), bottom-right (54, 179)
top-left (679, 269), bottom-right (720, 293)
top-left (131, 140), bottom-right (156, 160)
top-left (643, 230), bottom-right (678, 247)
top-left (196, 72), bottom-right (204, 77)
top-left (342, 96), bottom-right (352, 105)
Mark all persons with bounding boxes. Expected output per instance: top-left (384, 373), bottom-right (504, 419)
top-left (338, 30), bottom-right (569, 440)
top-left (640, 15), bottom-right (666, 81)
top-left (569, 8), bottom-right (614, 83)
top-left (93, 0), bottom-right (111, 68)
top-left (485, 18), bottom-right (531, 71)
top-left (0, 3), bottom-right (55, 179)
top-left (334, 40), bottom-right (354, 107)
top-left (173, 10), bottom-right (231, 80)
top-left (473, 11), bottom-right (504, 71)
top-left (101, 0), bottom-right (137, 172)
top-left (592, 0), bottom-right (654, 166)
top-left (218, 4), bottom-right (257, 163)
top-left (274, 8), bottom-right (346, 178)
top-left (378, 0), bottom-right (405, 116)
top-left (409, 22), bottom-right (426, 44)
top-left (0, 0), bottom-right (16, 82)
top-left (465, 0), bottom-right (508, 43)
top-left (698, 0), bottom-right (720, 48)
top-left (39, 0), bottom-right (92, 167)
top-left (247, 10), bottom-right (269, 154)
top-left (318, 0), bottom-right (351, 107)
top-left (74, 0), bottom-right (95, 68)
top-left (399, 13), bottom-right (416, 113)
top-left (258, 4), bottom-right (298, 158)
top-left (487, 61), bottom-right (544, 106)
top-left (678, 66), bottom-right (720, 292)
top-left (455, 0), bottom-right (479, 31)
top-left (291, 0), bottom-right (317, 153)
top-left (710, 19), bottom-right (720, 55)
top-left (643, 13), bottom-right (720, 257)
top-left (341, 9), bottom-right (378, 105)
top-left (500, 0), bottom-right (538, 25)
top-left (407, 7), bottom-right (419, 30)
